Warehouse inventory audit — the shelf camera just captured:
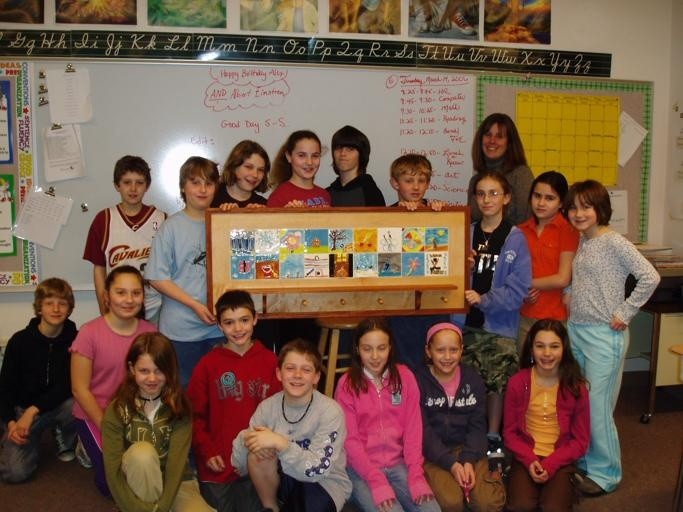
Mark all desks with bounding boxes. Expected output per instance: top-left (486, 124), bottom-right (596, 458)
top-left (624, 265), bottom-right (683, 423)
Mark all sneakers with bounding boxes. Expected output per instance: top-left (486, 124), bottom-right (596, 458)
top-left (579, 476), bottom-right (603, 500)
top-left (74, 432), bottom-right (92, 470)
top-left (49, 426), bottom-right (72, 462)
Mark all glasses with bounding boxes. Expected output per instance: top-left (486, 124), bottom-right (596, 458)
top-left (476, 191), bottom-right (506, 197)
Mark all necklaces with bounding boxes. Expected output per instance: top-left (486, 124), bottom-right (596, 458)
top-left (137, 392), bottom-right (164, 404)
top-left (478, 220), bottom-right (505, 247)
top-left (282, 395), bottom-right (315, 425)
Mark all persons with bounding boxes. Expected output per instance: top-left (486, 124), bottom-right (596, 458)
top-left (266, 131), bottom-right (331, 209)
top-left (415, 320), bottom-right (507, 512)
top-left (100, 331), bottom-right (193, 512)
top-left (0, 279), bottom-right (79, 485)
top-left (560, 179), bottom-right (661, 496)
top-left (503, 318), bottom-right (590, 512)
top-left (189, 290), bottom-right (283, 511)
top-left (83, 155), bottom-right (168, 328)
top-left (246, 340), bottom-right (352, 512)
top-left (417, 0), bottom-right (474, 36)
top-left (390, 154), bottom-right (450, 213)
top-left (335, 318), bottom-right (441, 511)
top-left (450, 170), bottom-right (532, 460)
top-left (516, 171), bottom-right (580, 359)
top-left (70, 266), bottom-right (156, 496)
top-left (143, 157), bottom-right (238, 386)
top-left (211, 140), bottom-right (271, 209)
top-left (448, 114), bottom-right (534, 225)
top-left (324, 125), bottom-right (387, 208)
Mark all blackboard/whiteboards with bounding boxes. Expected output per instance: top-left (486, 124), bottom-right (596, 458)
top-left (0, 60), bottom-right (476, 291)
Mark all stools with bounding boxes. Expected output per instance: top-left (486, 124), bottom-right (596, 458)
top-left (312, 322), bottom-right (362, 398)
